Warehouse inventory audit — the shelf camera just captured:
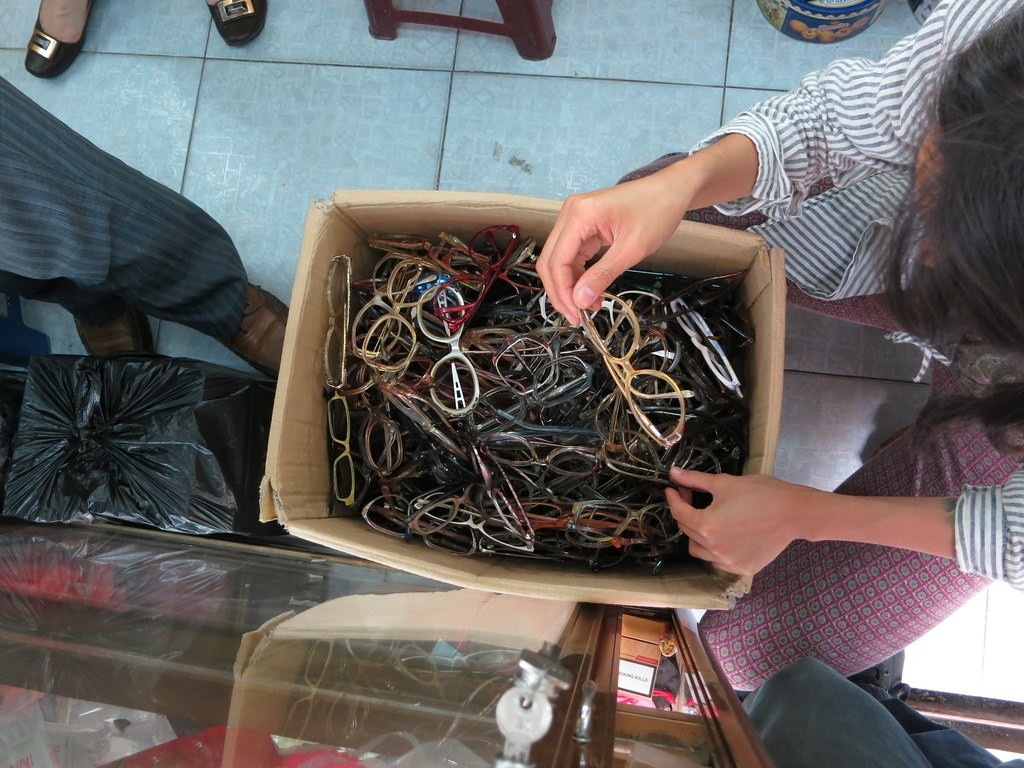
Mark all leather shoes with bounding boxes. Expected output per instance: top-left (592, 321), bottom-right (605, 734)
top-left (206, 0), bottom-right (267, 46)
top-left (73, 311), bottom-right (154, 357)
top-left (225, 284), bottom-right (290, 378)
top-left (25, 0), bottom-right (93, 78)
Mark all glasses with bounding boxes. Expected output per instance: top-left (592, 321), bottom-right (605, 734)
top-left (274, 639), bottom-right (520, 756)
top-left (319, 224), bottom-right (752, 580)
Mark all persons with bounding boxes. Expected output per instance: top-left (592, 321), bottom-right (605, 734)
top-left (24, 0), bottom-right (268, 79)
top-left (533, 0), bottom-right (1023, 693)
top-left (0, 75), bottom-right (290, 382)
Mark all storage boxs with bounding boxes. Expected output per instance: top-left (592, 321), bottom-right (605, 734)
top-left (220, 588), bottom-right (601, 767)
top-left (257, 190), bottom-right (786, 611)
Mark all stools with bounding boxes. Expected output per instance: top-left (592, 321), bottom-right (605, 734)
top-left (363, 0), bottom-right (557, 62)
